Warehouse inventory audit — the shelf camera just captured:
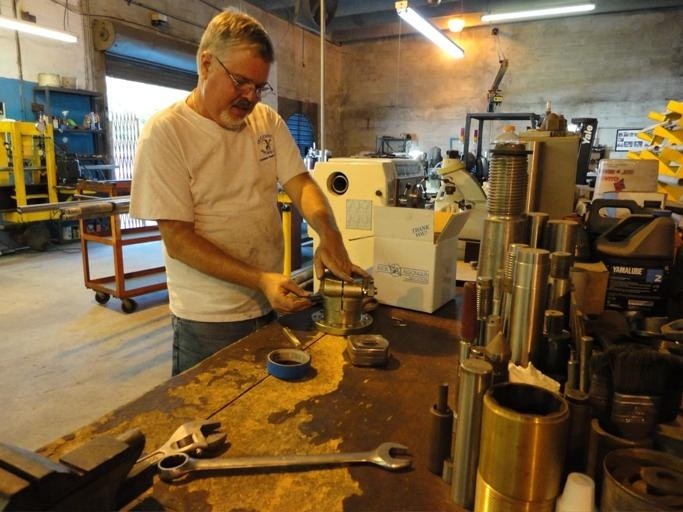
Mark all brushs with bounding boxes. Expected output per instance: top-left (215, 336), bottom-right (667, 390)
top-left (588, 345), bottom-right (683, 443)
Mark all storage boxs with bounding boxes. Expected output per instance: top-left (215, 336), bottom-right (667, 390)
top-left (372, 206), bottom-right (472, 314)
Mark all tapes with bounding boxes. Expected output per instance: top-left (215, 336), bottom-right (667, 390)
top-left (267, 348), bottom-right (311, 380)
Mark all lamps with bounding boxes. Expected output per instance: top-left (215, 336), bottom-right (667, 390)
top-left (0, 0), bottom-right (79, 43)
top-left (478, 1), bottom-right (595, 23)
top-left (395, 0), bottom-right (465, 58)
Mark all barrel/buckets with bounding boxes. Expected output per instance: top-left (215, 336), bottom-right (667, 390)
top-left (591, 157), bottom-right (662, 203)
top-left (597, 211), bottom-right (678, 318)
top-left (586, 197), bottom-right (664, 260)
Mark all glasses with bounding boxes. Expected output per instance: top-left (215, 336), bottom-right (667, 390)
top-left (215, 56), bottom-right (273, 98)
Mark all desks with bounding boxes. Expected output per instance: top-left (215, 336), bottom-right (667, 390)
top-left (33, 286), bottom-right (474, 511)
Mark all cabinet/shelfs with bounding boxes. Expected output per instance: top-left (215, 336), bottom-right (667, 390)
top-left (32, 86), bottom-right (119, 180)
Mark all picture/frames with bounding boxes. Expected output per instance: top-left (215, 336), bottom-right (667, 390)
top-left (615, 129), bottom-right (644, 152)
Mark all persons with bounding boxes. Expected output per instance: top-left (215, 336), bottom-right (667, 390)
top-left (128, 6), bottom-right (369, 377)
